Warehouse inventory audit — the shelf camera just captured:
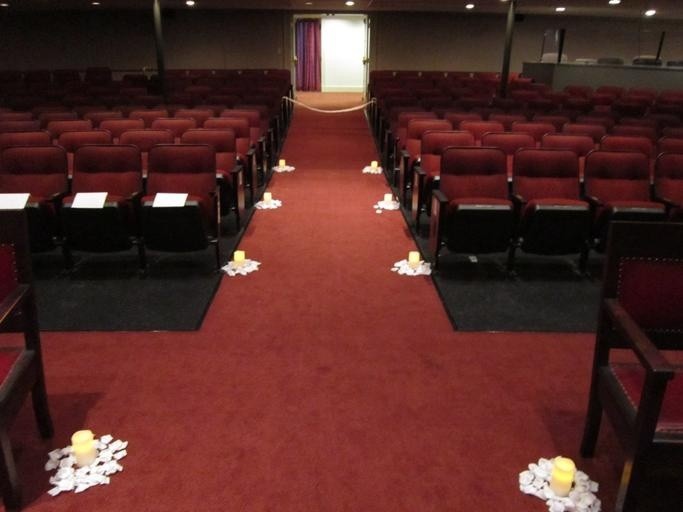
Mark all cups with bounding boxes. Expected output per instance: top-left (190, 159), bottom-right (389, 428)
top-left (263, 192), bottom-right (271, 204)
top-left (233, 250), bottom-right (245, 269)
top-left (551, 456), bottom-right (575, 496)
top-left (278, 159), bottom-right (285, 169)
top-left (384, 194), bottom-right (392, 205)
top-left (408, 251), bottom-right (420, 269)
top-left (70, 430), bottom-right (97, 468)
top-left (370, 160), bottom-right (378, 170)
